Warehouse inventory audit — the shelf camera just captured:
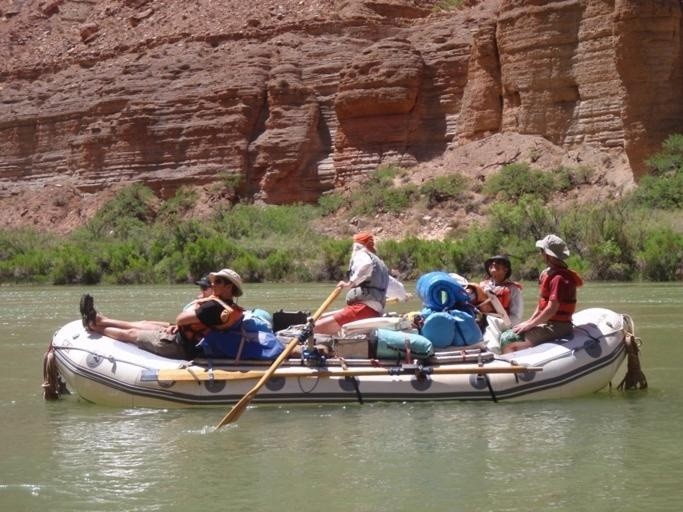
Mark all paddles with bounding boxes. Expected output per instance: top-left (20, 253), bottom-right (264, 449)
top-left (157, 365), bottom-right (543, 381)
top-left (214, 286), bottom-right (342, 429)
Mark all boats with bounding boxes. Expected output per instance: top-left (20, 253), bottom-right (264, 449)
top-left (43, 306), bottom-right (633, 407)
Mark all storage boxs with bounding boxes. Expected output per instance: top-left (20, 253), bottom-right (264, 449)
top-left (332, 336), bottom-right (369, 359)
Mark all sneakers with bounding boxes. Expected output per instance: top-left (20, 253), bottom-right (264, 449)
top-left (80, 294), bottom-right (98, 330)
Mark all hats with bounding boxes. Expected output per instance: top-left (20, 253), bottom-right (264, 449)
top-left (536, 235), bottom-right (569, 259)
top-left (354, 231), bottom-right (375, 253)
top-left (195, 268), bottom-right (243, 297)
top-left (484, 255), bottom-right (512, 278)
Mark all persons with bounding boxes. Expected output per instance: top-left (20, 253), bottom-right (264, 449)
top-left (79, 268), bottom-right (245, 359)
top-left (464, 254), bottom-right (525, 355)
top-left (310, 232), bottom-right (410, 334)
top-left (499, 233), bottom-right (584, 354)
